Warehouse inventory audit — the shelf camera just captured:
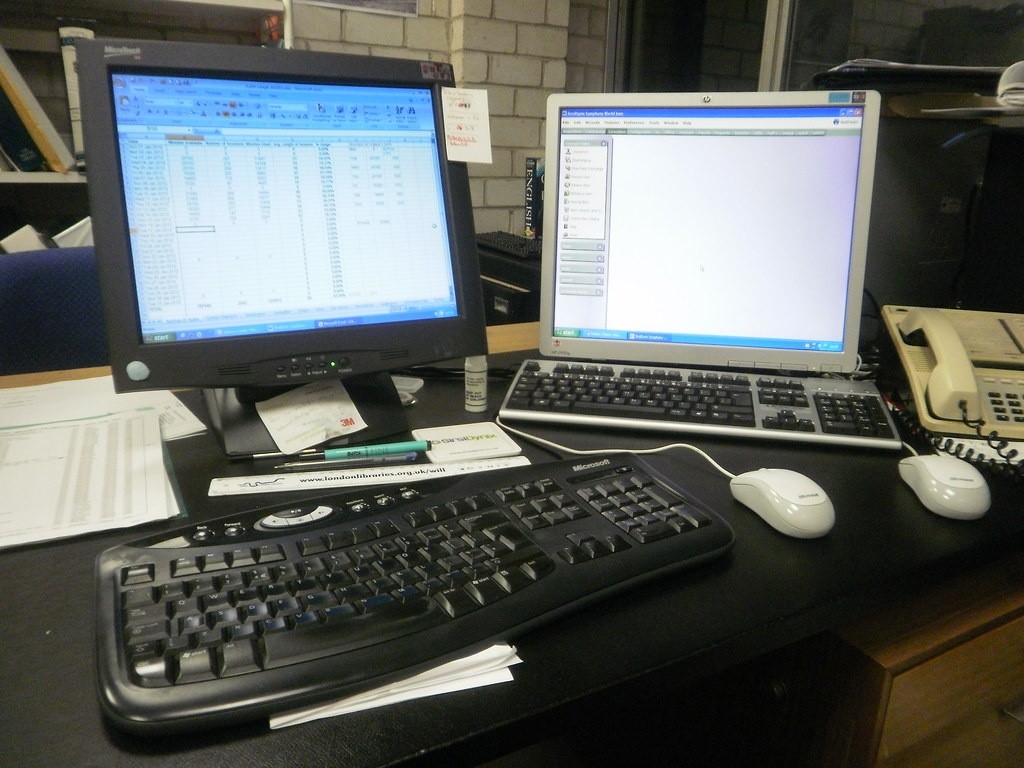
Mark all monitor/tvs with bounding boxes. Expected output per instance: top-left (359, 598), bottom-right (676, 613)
top-left (539, 88), bottom-right (900, 376)
top-left (73, 34), bottom-right (491, 397)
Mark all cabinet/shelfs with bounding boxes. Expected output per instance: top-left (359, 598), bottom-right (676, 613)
top-left (795, 602), bottom-right (1024, 768)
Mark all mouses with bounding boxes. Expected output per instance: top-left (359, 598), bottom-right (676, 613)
top-left (730, 467), bottom-right (837, 538)
top-left (899, 453), bottom-right (994, 520)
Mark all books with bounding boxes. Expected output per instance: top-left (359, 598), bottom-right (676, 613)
top-left (522, 155), bottom-right (544, 242)
top-left (2, 15), bottom-right (98, 175)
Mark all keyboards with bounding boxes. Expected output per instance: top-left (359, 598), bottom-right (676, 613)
top-left (499, 358), bottom-right (906, 451)
top-left (91, 445), bottom-right (737, 734)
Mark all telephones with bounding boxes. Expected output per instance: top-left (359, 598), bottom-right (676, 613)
top-left (880, 304), bottom-right (1024, 440)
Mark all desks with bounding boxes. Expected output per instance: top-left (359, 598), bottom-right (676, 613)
top-left (0, 223), bottom-right (1024, 767)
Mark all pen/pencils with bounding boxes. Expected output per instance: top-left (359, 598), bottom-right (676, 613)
top-left (273, 450), bottom-right (420, 471)
top-left (226, 449), bottom-right (318, 460)
top-left (300, 439), bottom-right (433, 460)
